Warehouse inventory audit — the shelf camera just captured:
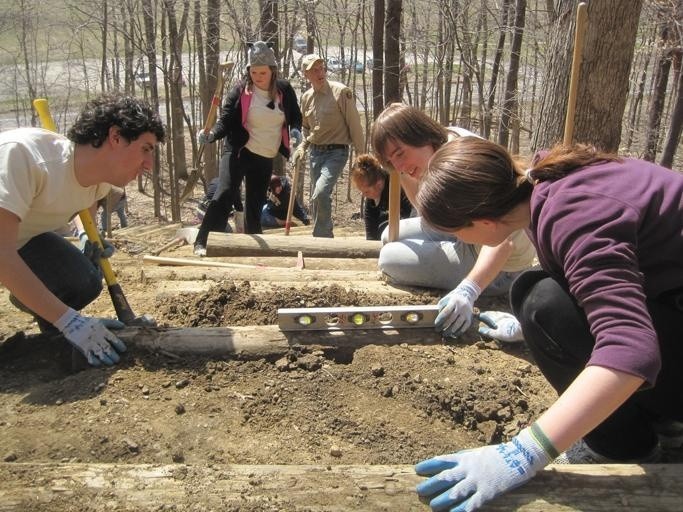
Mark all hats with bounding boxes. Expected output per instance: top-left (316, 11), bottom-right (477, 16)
top-left (269, 175), bottom-right (281, 187)
top-left (301, 54), bottom-right (324, 72)
top-left (245, 41), bottom-right (278, 72)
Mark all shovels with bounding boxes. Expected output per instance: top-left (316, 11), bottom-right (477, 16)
top-left (181, 61), bottom-right (235, 205)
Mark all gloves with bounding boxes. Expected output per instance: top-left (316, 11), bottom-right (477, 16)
top-left (292, 147), bottom-right (304, 167)
top-left (198, 128), bottom-right (215, 144)
top-left (290, 127), bottom-right (303, 148)
top-left (78, 231), bottom-right (115, 267)
top-left (415, 421), bottom-right (560, 512)
top-left (432, 277), bottom-right (482, 339)
top-left (52, 307), bottom-right (127, 368)
top-left (478, 310), bottom-right (525, 343)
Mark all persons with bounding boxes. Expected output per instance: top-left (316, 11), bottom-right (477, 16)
top-left (292, 51), bottom-right (367, 239)
top-left (413, 132), bottom-right (683, 512)
top-left (0, 89), bottom-right (168, 367)
top-left (351, 152), bottom-right (417, 240)
top-left (193, 41), bottom-right (303, 259)
top-left (195, 176), bottom-right (243, 235)
top-left (260, 176), bottom-right (311, 226)
top-left (371, 99), bottom-right (537, 338)
top-left (89, 187), bottom-right (128, 232)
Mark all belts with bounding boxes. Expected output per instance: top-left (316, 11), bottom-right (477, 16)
top-left (309, 142), bottom-right (349, 152)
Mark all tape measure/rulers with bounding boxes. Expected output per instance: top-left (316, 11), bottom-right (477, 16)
top-left (278, 305), bottom-right (439, 331)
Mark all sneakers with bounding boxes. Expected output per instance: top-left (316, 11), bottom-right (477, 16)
top-left (196, 223), bottom-right (202, 229)
top-left (193, 244), bottom-right (207, 256)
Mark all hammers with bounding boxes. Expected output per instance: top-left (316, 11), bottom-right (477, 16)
top-left (32, 99), bottom-right (157, 328)
